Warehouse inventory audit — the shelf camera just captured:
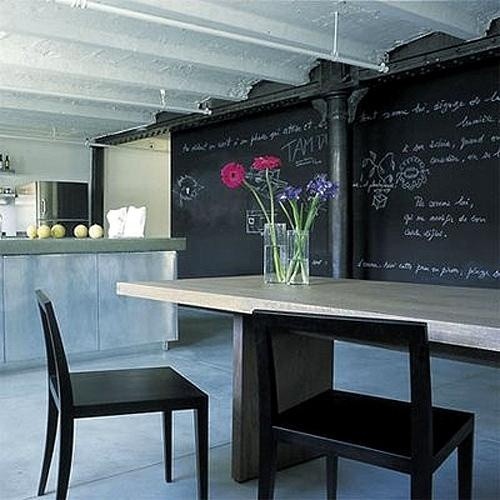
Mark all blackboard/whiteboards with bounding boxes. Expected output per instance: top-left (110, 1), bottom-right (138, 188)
top-left (170, 47), bottom-right (500, 290)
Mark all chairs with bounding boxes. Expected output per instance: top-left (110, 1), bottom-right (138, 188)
top-left (33, 289), bottom-right (209, 500)
top-left (251, 309), bottom-right (475, 500)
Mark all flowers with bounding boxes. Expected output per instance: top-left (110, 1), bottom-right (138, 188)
top-left (260, 170), bottom-right (341, 286)
top-left (219, 154), bottom-right (286, 282)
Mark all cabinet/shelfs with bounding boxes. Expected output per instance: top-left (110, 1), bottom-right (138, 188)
top-left (0, 169), bottom-right (17, 198)
top-left (0, 250), bottom-right (180, 369)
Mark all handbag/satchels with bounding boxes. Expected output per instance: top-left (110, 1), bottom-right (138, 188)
top-left (106, 205), bottom-right (148, 238)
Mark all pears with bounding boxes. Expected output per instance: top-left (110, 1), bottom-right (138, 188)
top-left (37, 224), bottom-right (50, 239)
top-left (89, 223), bottom-right (102, 239)
top-left (27, 224), bottom-right (37, 239)
top-left (73, 224), bottom-right (87, 238)
top-left (50, 224), bottom-right (66, 238)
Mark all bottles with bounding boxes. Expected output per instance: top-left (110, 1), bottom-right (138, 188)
top-left (0, 154), bottom-right (10, 170)
top-left (1, 187), bottom-right (11, 193)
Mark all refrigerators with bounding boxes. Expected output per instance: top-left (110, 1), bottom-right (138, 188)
top-left (14, 181), bottom-right (89, 237)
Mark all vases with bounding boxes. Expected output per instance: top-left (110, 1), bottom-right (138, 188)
top-left (286, 229), bottom-right (310, 287)
top-left (263, 222), bottom-right (287, 285)
